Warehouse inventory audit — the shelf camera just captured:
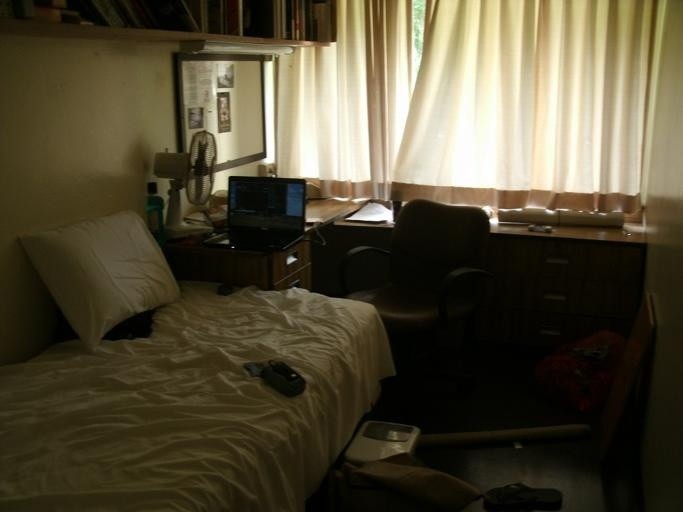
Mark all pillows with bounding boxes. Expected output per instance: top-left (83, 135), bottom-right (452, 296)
top-left (1, 205), bottom-right (180, 369)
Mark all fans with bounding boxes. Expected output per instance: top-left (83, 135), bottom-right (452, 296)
top-left (150, 128), bottom-right (217, 241)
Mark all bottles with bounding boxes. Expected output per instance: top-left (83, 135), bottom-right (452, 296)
top-left (144, 182), bottom-right (165, 239)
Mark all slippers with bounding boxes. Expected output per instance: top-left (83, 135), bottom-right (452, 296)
top-left (482, 482), bottom-right (563, 511)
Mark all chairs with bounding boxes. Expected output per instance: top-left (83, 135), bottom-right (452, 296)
top-left (337, 199), bottom-right (496, 412)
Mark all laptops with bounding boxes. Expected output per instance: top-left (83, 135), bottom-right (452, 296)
top-left (202, 175), bottom-right (306, 252)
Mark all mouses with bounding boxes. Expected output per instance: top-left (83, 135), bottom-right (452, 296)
top-left (217, 283), bottom-right (234, 295)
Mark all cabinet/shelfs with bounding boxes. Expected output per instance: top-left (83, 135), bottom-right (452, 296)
top-left (185, 199), bottom-right (368, 292)
top-left (334, 221), bottom-right (644, 358)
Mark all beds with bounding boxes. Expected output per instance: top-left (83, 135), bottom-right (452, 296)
top-left (0, 289), bottom-right (377, 509)
